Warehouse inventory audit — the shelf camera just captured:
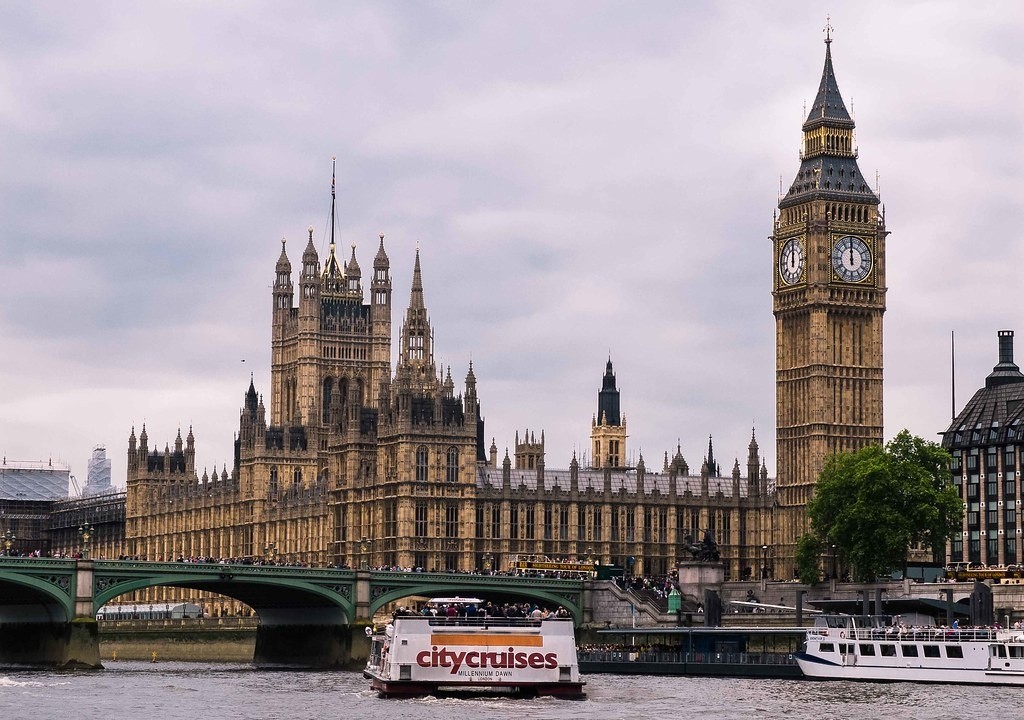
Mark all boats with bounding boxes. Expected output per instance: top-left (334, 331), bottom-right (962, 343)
top-left (364, 596), bottom-right (590, 699)
top-left (794, 618), bottom-right (1023, 688)
top-left (576, 624), bottom-right (809, 681)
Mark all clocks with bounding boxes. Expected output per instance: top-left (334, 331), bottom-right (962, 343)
top-left (832, 235), bottom-right (873, 282)
top-left (780, 239), bottom-right (803, 283)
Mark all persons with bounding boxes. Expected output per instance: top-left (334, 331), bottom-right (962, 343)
top-left (0, 548), bottom-right (1024, 664)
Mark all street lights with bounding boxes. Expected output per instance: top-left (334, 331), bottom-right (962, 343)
top-left (356, 536), bottom-right (371, 570)
top-left (0, 529), bottom-right (16, 555)
top-left (482, 551), bottom-right (495, 574)
top-left (762, 545), bottom-right (769, 579)
top-left (264, 542), bottom-right (278, 565)
top-left (831, 543), bottom-right (837, 579)
top-left (78, 522), bottom-right (95, 560)
top-left (921, 529), bottom-right (931, 583)
